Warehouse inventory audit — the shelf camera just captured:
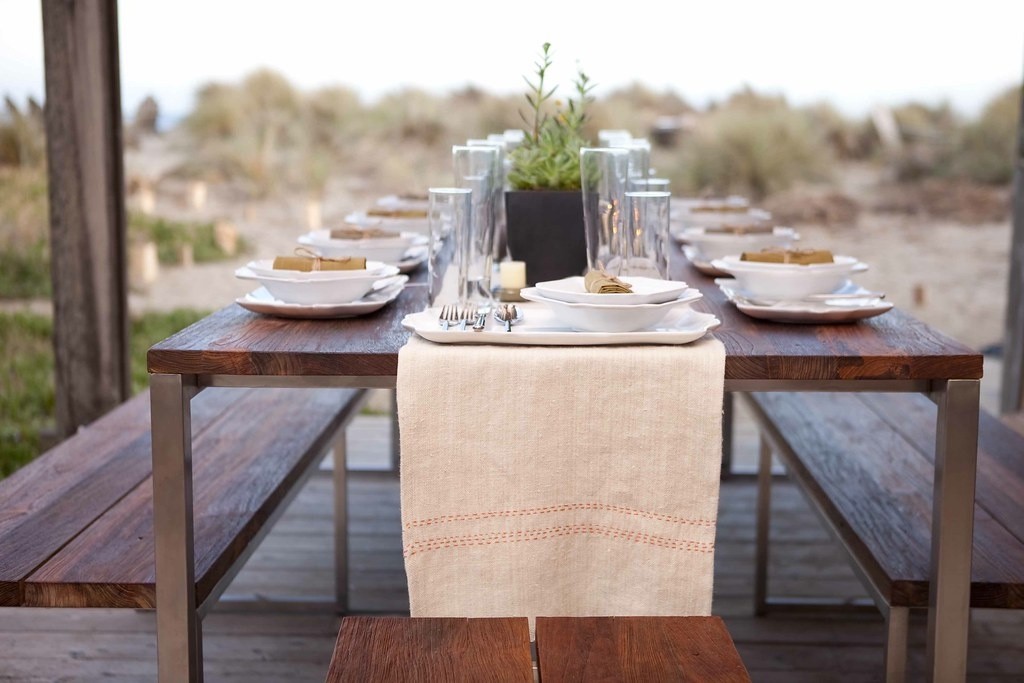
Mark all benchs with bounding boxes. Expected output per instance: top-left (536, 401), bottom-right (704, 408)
top-left (0, 378), bottom-right (381, 683)
top-left (742, 389), bottom-right (1021, 667)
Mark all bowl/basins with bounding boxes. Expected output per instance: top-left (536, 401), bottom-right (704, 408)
top-left (234, 203), bottom-right (434, 304)
top-left (674, 198), bottom-right (869, 299)
top-left (519, 287), bottom-right (704, 333)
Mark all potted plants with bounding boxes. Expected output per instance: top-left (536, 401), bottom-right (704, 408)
top-left (502, 44), bottom-right (601, 292)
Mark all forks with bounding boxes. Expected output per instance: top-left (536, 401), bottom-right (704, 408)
top-left (439, 304), bottom-right (479, 332)
top-left (733, 291), bottom-right (886, 306)
top-left (362, 285), bottom-right (389, 299)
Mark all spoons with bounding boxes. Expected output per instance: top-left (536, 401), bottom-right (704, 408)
top-left (494, 303), bottom-right (518, 332)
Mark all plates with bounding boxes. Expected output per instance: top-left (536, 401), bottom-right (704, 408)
top-left (673, 229), bottom-right (895, 321)
top-left (234, 226), bottom-right (449, 318)
top-left (723, 254), bottom-right (860, 269)
top-left (247, 259), bottom-right (386, 280)
top-left (535, 273), bottom-right (687, 305)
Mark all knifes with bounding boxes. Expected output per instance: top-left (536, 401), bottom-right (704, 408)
top-left (472, 306), bottom-right (491, 330)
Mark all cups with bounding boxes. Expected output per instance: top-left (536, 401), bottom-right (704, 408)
top-left (579, 129), bottom-right (672, 280)
top-left (425, 130), bottom-right (524, 305)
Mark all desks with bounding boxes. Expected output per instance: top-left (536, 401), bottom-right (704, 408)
top-left (143, 167), bottom-right (983, 683)
